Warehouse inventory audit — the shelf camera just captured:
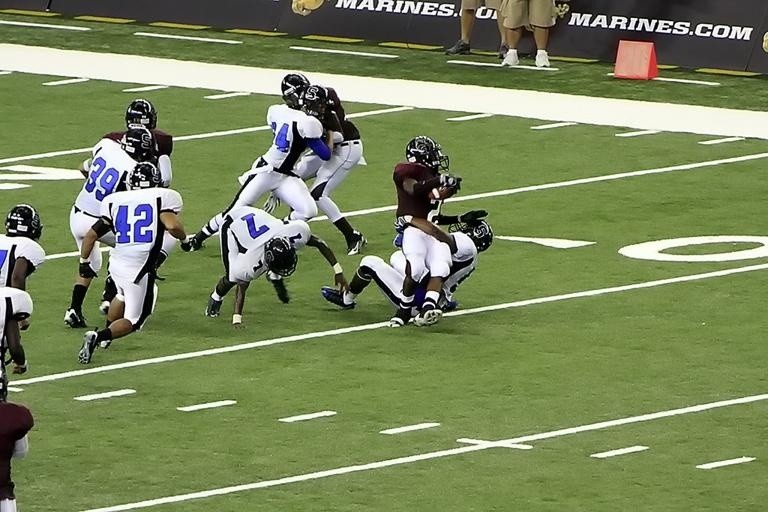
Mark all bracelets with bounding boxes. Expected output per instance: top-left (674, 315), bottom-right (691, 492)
top-left (15, 359), bottom-right (30, 369)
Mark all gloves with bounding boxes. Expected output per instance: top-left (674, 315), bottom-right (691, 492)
top-left (394, 214), bottom-right (415, 234)
top-left (76, 261), bottom-right (100, 280)
top-left (179, 237), bottom-right (191, 253)
top-left (304, 84), bottom-right (328, 102)
top-left (17, 317), bottom-right (30, 331)
top-left (12, 360), bottom-right (28, 374)
top-left (438, 174), bottom-right (463, 192)
top-left (460, 208), bottom-right (490, 227)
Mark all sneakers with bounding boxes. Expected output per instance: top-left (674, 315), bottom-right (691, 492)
top-left (445, 39), bottom-right (471, 56)
top-left (408, 303), bottom-right (444, 328)
top-left (345, 227), bottom-right (370, 257)
top-left (387, 305), bottom-right (413, 329)
top-left (264, 272), bottom-right (291, 305)
top-left (319, 283), bottom-right (357, 311)
top-left (77, 330), bottom-right (98, 365)
top-left (100, 339), bottom-right (111, 349)
top-left (533, 49), bottom-right (551, 68)
top-left (98, 300), bottom-right (111, 313)
top-left (497, 42), bottom-right (509, 59)
top-left (63, 307), bottom-right (88, 328)
top-left (188, 236), bottom-right (201, 251)
top-left (500, 49), bottom-right (519, 67)
top-left (204, 291), bottom-right (225, 319)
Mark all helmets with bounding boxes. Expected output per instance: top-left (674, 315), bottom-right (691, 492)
top-left (124, 97), bottom-right (158, 130)
top-left (404, 134), bottom-right (446, 169)
top-left (118, 123), bottom-right (161, 162)
top-left (0, 366), bottom-right (10, 400)
top-left (280, 71), bottom-right (310, 109)
top-left (127, 162), bottom-right (163, 190)
top-left (262, 235), bottom-right (299, 272)
top-left (459, 220), bottom-right (495, 252)
top-left (5, 203), bottom-right (44, 240)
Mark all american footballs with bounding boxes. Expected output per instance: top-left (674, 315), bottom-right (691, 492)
top-left (429, 183), bottom-right (455, 199)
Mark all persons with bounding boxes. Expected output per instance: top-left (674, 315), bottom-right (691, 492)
top-left (0, 368), bottom-right (35, 512)
top-left (0, 200), bottom-right (48, 352)
top-left (497, 0), bottom-right (559, 69)
top-left (0, 287), bottom-right (36, 402)
top-left (444, 0), bottom-right (509, 58)
